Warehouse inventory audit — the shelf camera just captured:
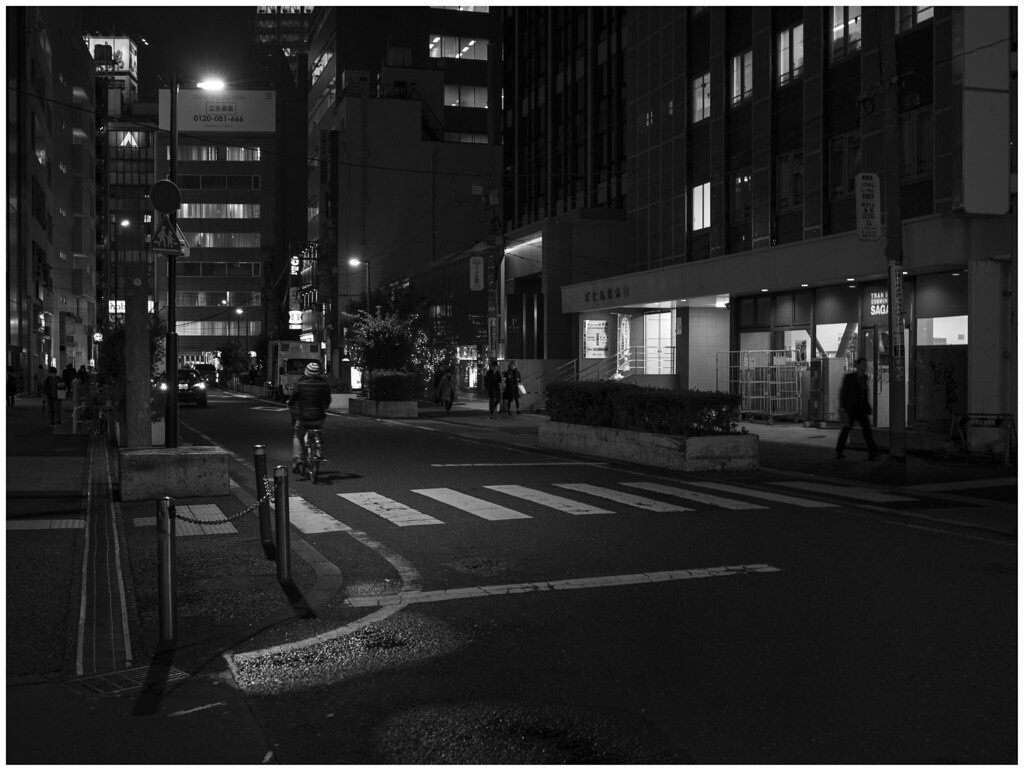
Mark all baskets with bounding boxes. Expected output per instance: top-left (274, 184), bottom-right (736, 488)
top-left (290, 410), bottom-right (297, 426)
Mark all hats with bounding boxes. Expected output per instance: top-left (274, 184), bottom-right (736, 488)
top-left (304, 362), bottom-right (320, 376)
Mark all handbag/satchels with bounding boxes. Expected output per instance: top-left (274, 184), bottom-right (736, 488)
top-left (57, 390), bottom-right (66, 399)
top-left (451, 391), bottom-right (457, 401)
top-left (517, 382), bottom-right (526, 394)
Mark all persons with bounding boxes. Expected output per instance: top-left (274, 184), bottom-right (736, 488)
top-left (502, 362), bottom-right (521, 415)
top-left (30, 364), bottom-right (89, 427)
top-left (7, 365), bottom-right (18, 408)
top-left (436, 369), bottom-right (459, 416)
top-left (286, 362), bottom-right (331, 473)
top-left (834, 357), bottom-right (880, 459)
top-left (487, 362), bottom-right (502, 419)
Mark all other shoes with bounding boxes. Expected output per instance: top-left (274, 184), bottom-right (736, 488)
top-left (56, 421), bottom-right (61, 427)
top-left (868, 451), bottom-right (880, 460)
top-left (292, 458), bottom-right (302, 473)
top-left (49, 421), bottom-right (55, 428)
top-left (837, 452), bottom-right (845, 459)
top-left (517, 410), bottom-right (522, 415)
top-left (508, 411), bottom-right (513, 415)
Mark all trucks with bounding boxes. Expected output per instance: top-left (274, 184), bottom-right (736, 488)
top-left (265, 339), bottom-right (321, 403)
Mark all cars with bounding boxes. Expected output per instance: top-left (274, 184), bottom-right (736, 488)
top-left (156, 367), bottom-right (208, 410)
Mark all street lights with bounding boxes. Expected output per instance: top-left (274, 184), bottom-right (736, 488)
top-left (347, 258), bottom-right (371, 314)
top-left (164, 76), bottom-right (229, 446)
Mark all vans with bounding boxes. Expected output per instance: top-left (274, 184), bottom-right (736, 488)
top-left (191, 363), bottom-right (216, 387)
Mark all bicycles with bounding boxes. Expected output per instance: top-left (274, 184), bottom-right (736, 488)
top-left (287, 400), bottom-right (328, 485)
